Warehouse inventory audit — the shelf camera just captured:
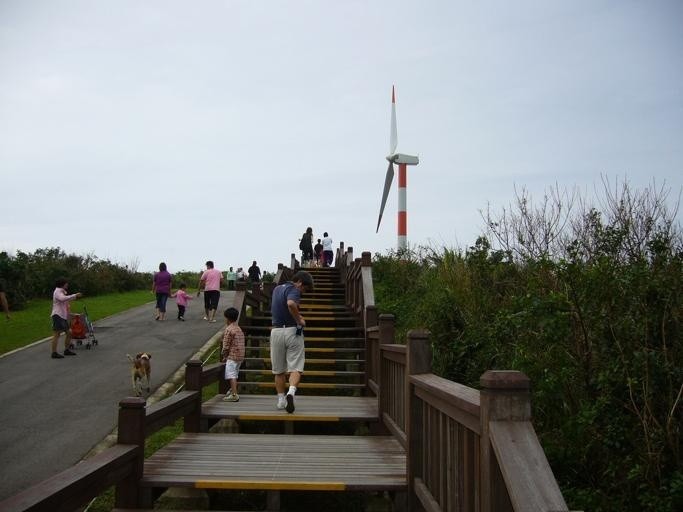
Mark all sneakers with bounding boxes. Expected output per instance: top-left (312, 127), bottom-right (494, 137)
top-left (285, 393), bottom-right (296, 414)
top-left (155, 313), bottom-right (217, 323)
top-left (222, 392), bottom-right (240, 402)
top-left (276, 400), bottom-right (286, 409)
top-left (63, 349), bottom-right (76, 356)
top-left (50, 352), bottom-right (64, 359)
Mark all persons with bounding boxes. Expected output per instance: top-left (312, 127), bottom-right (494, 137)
top-left (221, 307), bottom-right (245, 401)
top-left (226, 227), bottom-right (335, 290)
top-left (0, 278), bottom-right (10, 319)
top-left (51, 279), bottom-right (81, 358)
top-left (151, 262), bottom-right (171, 320)
top-left (170, 283), bottom-right (193, 322)
top-left (270, 269), bottom-right (314, 412)
top-left (197, 261), bottom-right (224, 322)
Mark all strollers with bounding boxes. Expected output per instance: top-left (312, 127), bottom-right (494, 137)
top-left (63, 301), bottom-right (102, 355)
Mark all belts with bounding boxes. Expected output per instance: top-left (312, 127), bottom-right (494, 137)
top-left (272, 323), bottom-right (297, 329)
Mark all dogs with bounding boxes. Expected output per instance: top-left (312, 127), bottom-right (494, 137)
top-left (126, 351), bottom-right (152, 397)
